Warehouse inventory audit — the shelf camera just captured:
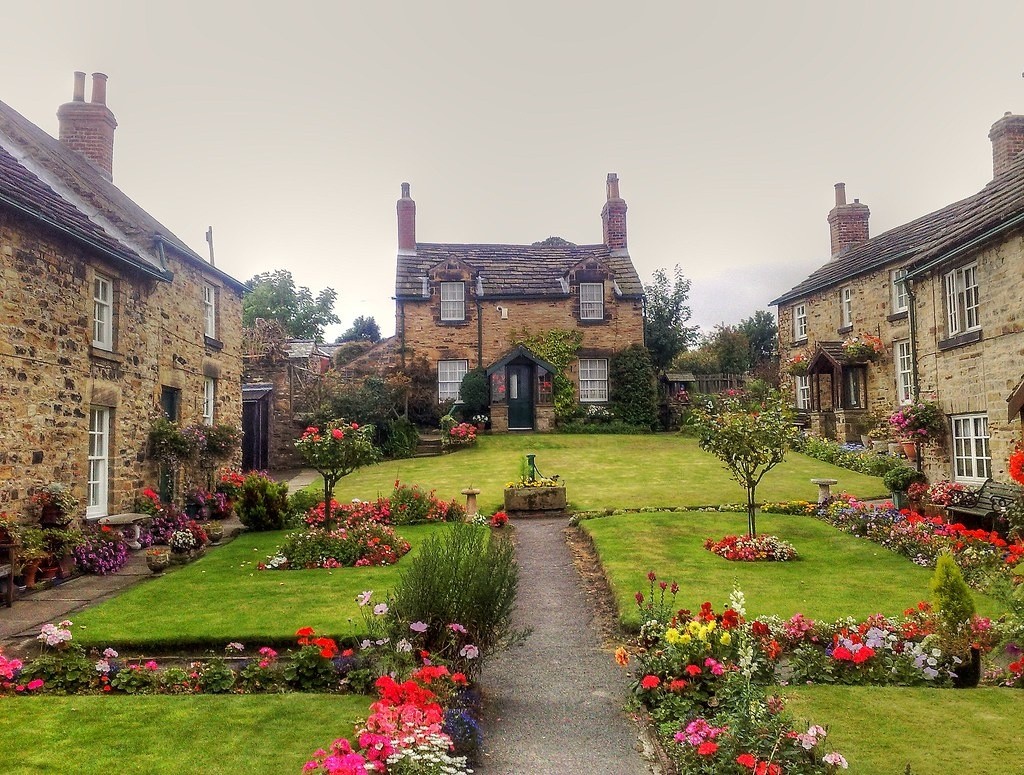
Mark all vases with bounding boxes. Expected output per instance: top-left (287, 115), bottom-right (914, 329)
top-left (902, 442), bottom-right (917, 462)
top-left (41, 507), bottom-right (59, 526)
top-left (477, 422), bottom-right (485, 430)
top-left (0, 526), bottom-right (8, 543)
top-left (907, 500), bottom-right (925, 512)
top-left (592, 418), bottom-right (602, 425)
top-left (145, 557), bottom-right (169, 574)
top-left (925, 504), bottom-right (946, 520)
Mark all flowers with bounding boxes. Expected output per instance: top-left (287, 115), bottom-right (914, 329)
top-left (147, 545), bottom-right (171, 564)
top-left (473, 414), bottom-right (489, 423)
top-left (0, 511), bottom-right (18, 542)
top-left (586, 404), bottom-right (615, 424)
top-left (927, 478), bottom-right (965, 506)
top-left (24, 482), bottom-right (81, 526)
top-left (903, 483), bottom-right (930, 500)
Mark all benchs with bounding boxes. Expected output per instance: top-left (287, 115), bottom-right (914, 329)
top-left (789, 409), bottom-right (808, 431)
top-left (945, 478), bottom-right (1023, 533)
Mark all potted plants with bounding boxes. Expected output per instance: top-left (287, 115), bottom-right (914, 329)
top-left (17, 526), bottom-right (49, 587)
top-left (202, 519), bottom-right (223, 546)
top-left (884, 467), bottom-right (927, 511)
top-left (884, 414), bottom-right (903, 457)
top-left (934, 549), bottom-right (982, 689)
top-left (869, 428), bottom-right (887, 453)
top-left (857, 409), bottom-right (884, 449)
top-left (40, 529), bottom-right (69, 581)
